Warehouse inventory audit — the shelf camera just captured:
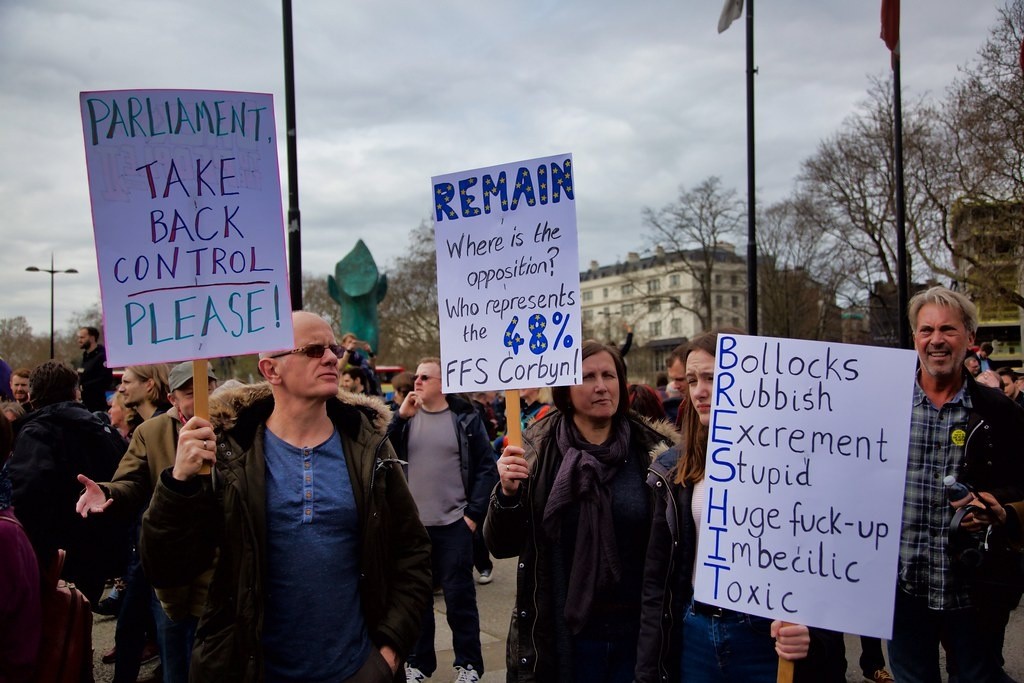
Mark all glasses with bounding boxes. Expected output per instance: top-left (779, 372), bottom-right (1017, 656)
top-left (268, 344), bottom-right (345, 359)
top-left (411, 375), bottom-right (441, 381)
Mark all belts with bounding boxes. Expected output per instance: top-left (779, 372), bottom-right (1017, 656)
top-left (694, 601), bottom-right (738, 618)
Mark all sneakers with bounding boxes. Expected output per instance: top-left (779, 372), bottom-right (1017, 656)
top-left (405, 664), bottom-right (427, 683)
top-left (102, 646), bottom-right (116, 663)
top-left (862, 669), bottom-right (894, 683)
top-left (92, 597), bottom-right (120, 616)
top-left (478, 565), bottom-right (493, 582)
top-left (453, 666), bottom-right (480, 683)
top-left (140, 645), bottom-right (160, 665)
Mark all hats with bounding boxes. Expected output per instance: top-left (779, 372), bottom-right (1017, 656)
top-left (168, 361), bottom-right (216, 391)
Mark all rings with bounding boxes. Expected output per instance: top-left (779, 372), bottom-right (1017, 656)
top-left (204, 441), bottom-right (207, 450)
top-left (507, 465), bottom-right (509, 471)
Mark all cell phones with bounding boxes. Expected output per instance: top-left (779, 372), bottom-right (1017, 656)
top-left (354, 341), bottom-right (364, 348)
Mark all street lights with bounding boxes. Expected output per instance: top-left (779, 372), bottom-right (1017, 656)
top-left (26, 252), bottom-right (78, 359)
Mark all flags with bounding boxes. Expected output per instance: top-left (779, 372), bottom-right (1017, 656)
top-left (880, 0), bottom-right (901, 70)
top-left (717, 0), bottom-right (744, 34)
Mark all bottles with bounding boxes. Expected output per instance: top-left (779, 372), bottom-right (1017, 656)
top-left (943, 475), bottom-right (992, 539)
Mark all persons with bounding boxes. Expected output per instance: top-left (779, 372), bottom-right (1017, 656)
top-left (0, 327), bottom-right (174, 683)
top-left (859, 635), bottom-right (894, 683)
top-left (76, 360), bottom-right (218, 683)
top-left (631, 327), bottom-right (847, 683)
top-left (964, 342), bottom-right (1024, 409)
top-left (386, 357), bottom-right (500, 683)
top-left (338, 334), bottom-right (415, 410)
top-left (887, 286), bottom-right (1024, 683)
top-left (956, 492), bottom-right (1024, 552)
top-left (139, 310), bottom-right (432, 683)
top-left (432, 387), bottom-right (554, 595)
top-left (482, 340), bottom-right (681, 683)
top-left (606, 324), bottom-right (688, 428)
